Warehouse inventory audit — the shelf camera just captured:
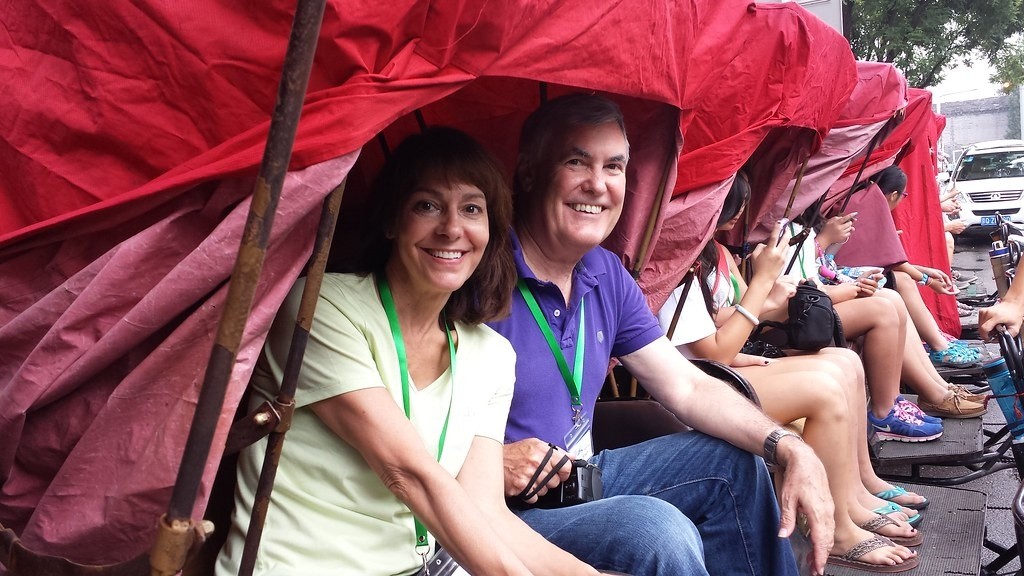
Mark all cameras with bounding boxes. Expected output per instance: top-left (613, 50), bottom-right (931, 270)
top-left (536, 460), bottom-right (604, 510)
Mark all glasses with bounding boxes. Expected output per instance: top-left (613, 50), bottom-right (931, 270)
top-left (897, 192), bottom-right (908, 198)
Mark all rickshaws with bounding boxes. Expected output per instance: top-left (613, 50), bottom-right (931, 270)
top-left (0, 0), bottom-right (1024, 576)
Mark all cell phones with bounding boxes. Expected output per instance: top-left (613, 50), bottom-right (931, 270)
top-left (845, 211), bottom-right (858, 222)
top-left (776, 218), bottom-right (788, 246)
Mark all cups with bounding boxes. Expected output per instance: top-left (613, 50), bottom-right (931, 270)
top-left (988, 241), bottom-right (1011, 303)
top-left (989, 230), bottom-right (1003, 241)
top-left (982, 357), bottom-right (1024, 440)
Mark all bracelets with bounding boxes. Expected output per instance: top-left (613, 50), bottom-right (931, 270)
top-left (815, 238), bottom-right (823, 258)
top-left (763, 430), bottom-right (806, 467)
top-left (735, 304), bottom-right (760, 325)
top-left (926, 277), bottom-right (933, 286)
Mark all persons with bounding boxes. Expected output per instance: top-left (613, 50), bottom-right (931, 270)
top-left (706, 175), bottom-right (931, 526)
top-left (922, 148), bottom-right (979, 289)
top-left (978, 248), bottom-right (1024, 347)
top-left (480, 89), bottom-right (837, 575)
top-left (657, 221), bottom-right (924, 573)
top-left (814, 212), bottom-right (988, 418)
top-left (780, 220), bottom-right (945, 443)
top-left (212, 124), bottom-right (604, 576)
top-left (869, 164), bottom-right (984, 368)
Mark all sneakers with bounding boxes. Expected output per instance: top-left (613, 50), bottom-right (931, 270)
top-left (868, 404), bottom-right (944, 442)
top-left (955, 280), bottom-right (970, 289)
top-left (948, 382), bottom-right (986, 403)
top-left (894, 394), bottom-right (943, 424)
top-left (957, 300), bottom-right (974, 309)
top-left (917, 389), bottom-right (987, 418)
top-left (929, 345), bottom-right (977, 367)
top-left (957, 307), bottom-right (972, 316)
top-left (949, 343), bottom-right (984, 362)
top-left (956, 273), bottom-right (978, 282)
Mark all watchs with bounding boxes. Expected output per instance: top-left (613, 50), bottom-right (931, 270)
top-left (917, 273), bottom-right (928, 286)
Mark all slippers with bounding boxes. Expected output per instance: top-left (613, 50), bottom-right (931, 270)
top-left (872, 500), bottom-right (924, 524)
top-left (826, 531), bottom-right (918, 572)
top-left (872, 484), bottom-right (930, 509)
top-left (856, 511), bottom-right (923, 546)
